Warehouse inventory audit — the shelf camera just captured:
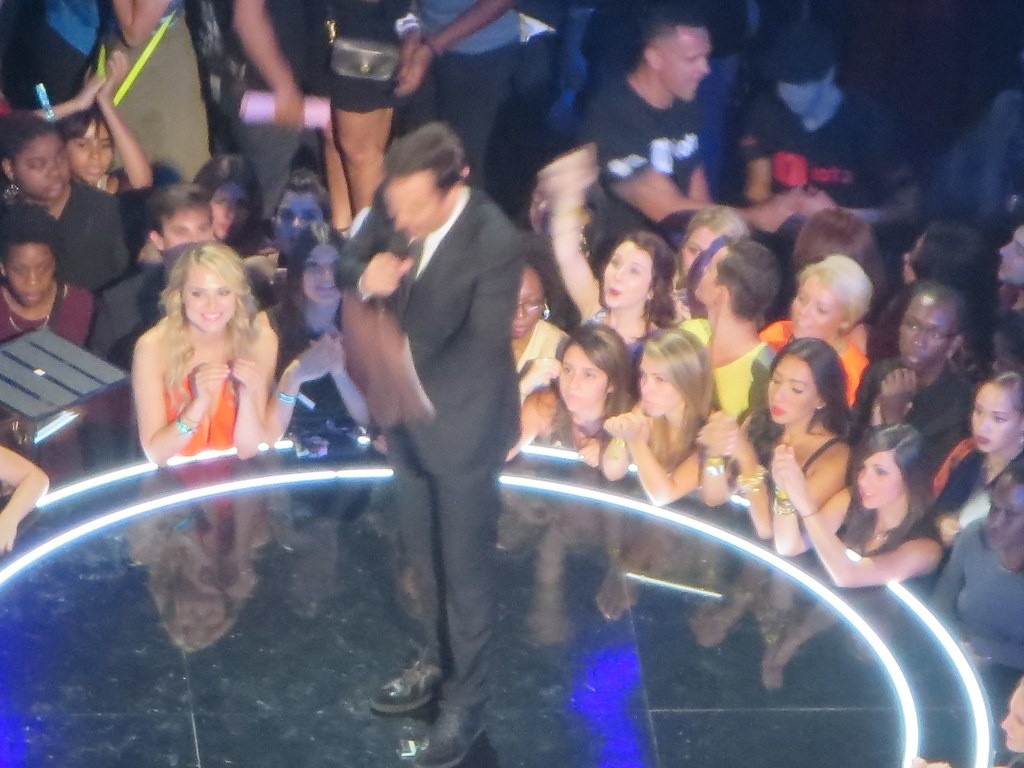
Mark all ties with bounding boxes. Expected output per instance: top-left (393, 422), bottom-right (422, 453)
top-left (395, 237), bottom-right (424, 321)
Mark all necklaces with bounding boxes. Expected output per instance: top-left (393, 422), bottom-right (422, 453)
top-left (869, 527), bottom-right (888, 542)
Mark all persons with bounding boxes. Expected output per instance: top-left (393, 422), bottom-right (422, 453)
top-left (1, 0), bottom-right (1024, 768)
top-left (494, 456), bottom-right (592, 645)
top-left (334, 118), bottom-right (526, 767)
top-left (596, 476), bottom-right (697, 623)
top-left (678, 515), bottom-right (774, 645)
top-left (757, 538), bottom-right (862, 691)
top-left (124, 447), bottom-right (286, 654)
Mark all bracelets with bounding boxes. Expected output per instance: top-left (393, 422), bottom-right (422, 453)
top-left (704, 453), bottom-right (726, 477)
top-left (393, 12), bottom-right (421, 36)
top-left (764, 603), bottom-right (793, 643)
top-left (608, 439), bottom-right (628, 459)
top-left (425, 39), bottom-right (441, 63)
top-left (737, 464), bottom-right (768, 492)
top-left (774, 483), bottom-right (797, 501)
top-left (173, 416), bottom-right (196, 435)
top-left (275, 390), bottom-right (298, 404)
top-left (773, 497), bottom-right (795, 517)
top-left (34, 84), bottom-right (56, 123)
top-left (606, 542), bottom-right (627, 561)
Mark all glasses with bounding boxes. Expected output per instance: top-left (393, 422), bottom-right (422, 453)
top-left (515, 299), bottom-right (546, 317)
top-left (899, 316), bottom-right (959, 347)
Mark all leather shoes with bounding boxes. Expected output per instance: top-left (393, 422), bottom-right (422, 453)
top-left (414, 705), bottom-right (485, 768)
top-left (368, 639), bottom-right (453, 712)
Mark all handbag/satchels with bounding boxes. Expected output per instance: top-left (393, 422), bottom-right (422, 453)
top-left (328, 39), bottom-right (401, 82)
